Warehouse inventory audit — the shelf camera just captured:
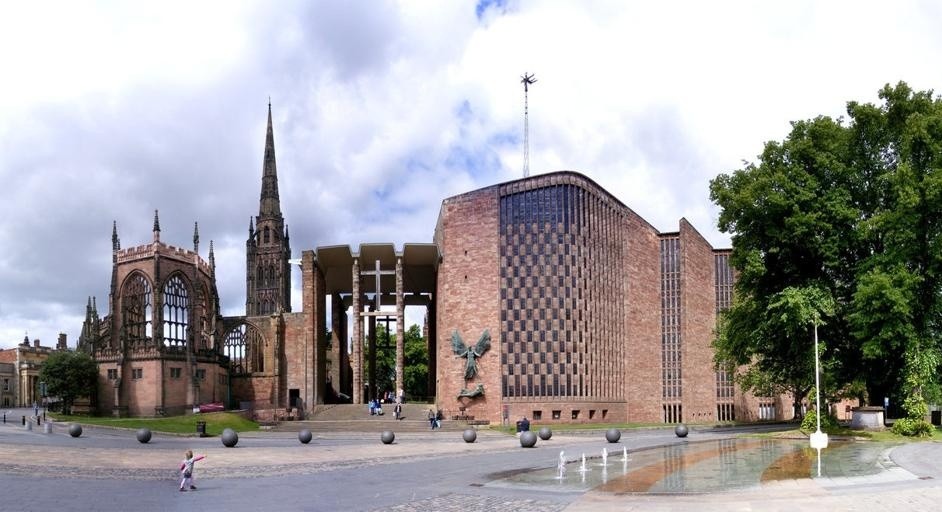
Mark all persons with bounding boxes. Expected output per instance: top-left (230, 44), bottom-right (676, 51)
top-left (521, 418), bottom-right (529, 432)
top-left (33, 400), bottom-right (38, 416)
top-left (454, 346), bottom-right (484, 399)
top-left (384, 391), bottom-right (395, 404)
top-left (428, 409), bottom-right (441, 430)
top-left (393, 403), bottom-right (401, 421)
top-left (179, 451), bottom-right (208, 491)
top-left (401, 388), bottom-right (406, 404)
top-left (369, 398), bottom-right (384, 416)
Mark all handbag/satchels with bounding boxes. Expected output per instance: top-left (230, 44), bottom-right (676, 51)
top-left (393, 411), bottom-right (396, 417)
top-left (184, 469), bottom-right (192, 478)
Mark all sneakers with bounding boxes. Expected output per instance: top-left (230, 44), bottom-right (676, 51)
top-left (189, 485), bottom-right (197, 488)
top-left (179, 487), bottom-right (187, 492)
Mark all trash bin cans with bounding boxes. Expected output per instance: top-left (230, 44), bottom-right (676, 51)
top-left (517, 420), bottom-right (529, 431)
top-left (197, 421), bottom-right (206, 437)
